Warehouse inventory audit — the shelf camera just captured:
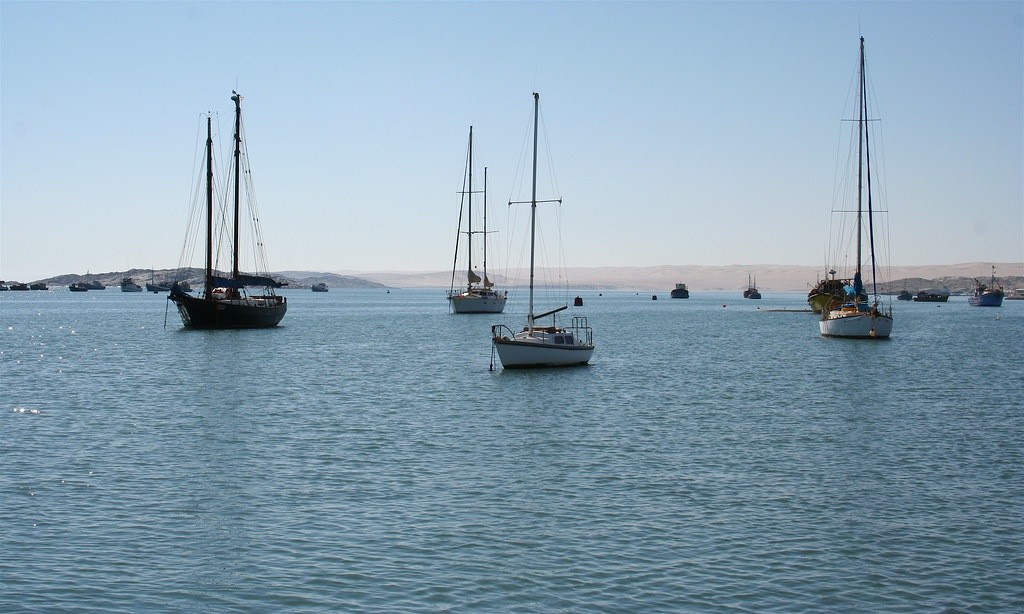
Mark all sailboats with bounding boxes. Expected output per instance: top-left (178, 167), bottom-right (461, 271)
top-left (164, 90), bottom-right (288, 330)
top-left (817, 36), bottom-right (895, 338)
top-left (744, 274), bottom-right (762, 300)
top-left (446, 126), bottom-right (507, 315)
top-left (486, 92), bottom-right (596, 373)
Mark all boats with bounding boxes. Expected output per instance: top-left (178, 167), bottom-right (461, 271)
top-left (968, 264), bottom-right (1005, 307)
top-left (669, 282), bottom-right (690, 299)
top-left (896, 288), bottom-right (913, 302)
top-left (806, 270), bottom-right (847, 313)
top-left (310, 282), bottom-right (328, 292)
top-left (912, 286), bottom-right (950, 304)
top-left (0, 275), bottom-right (191, 293)
